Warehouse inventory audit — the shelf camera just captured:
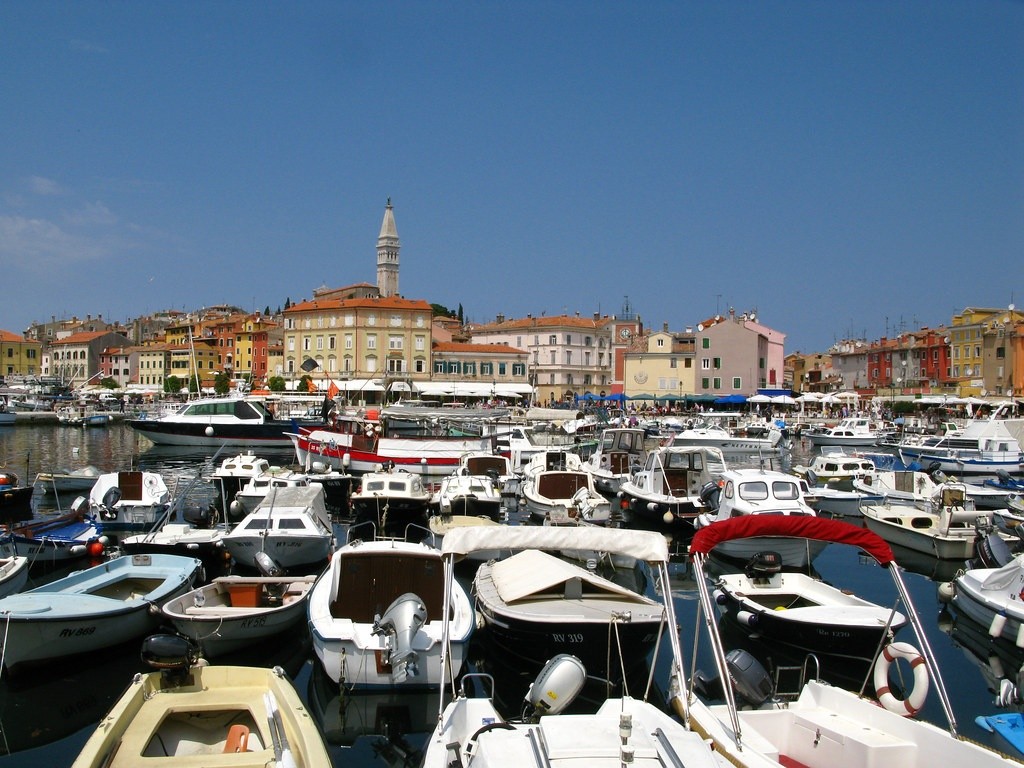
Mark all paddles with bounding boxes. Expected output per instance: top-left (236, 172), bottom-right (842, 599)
top-left (261, 688), bottom-right (298, 768)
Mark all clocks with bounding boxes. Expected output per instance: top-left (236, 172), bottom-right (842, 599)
top-left (620, 328), bottom-right (631, 340)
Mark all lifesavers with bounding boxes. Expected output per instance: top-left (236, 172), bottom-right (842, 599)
top-left (874, 642), bottom-right (931, 716)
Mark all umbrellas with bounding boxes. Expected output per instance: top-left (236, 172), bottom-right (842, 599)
top-left (422, 390), bottom-right (522, 398)
top-left (912, 397), bottom-right (1019, 408)
top-left (577, 392), bottom-right (861, 404)
top-left (82, 387), bottom-right (217, 394)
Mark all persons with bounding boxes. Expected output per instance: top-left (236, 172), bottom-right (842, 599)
top-left (452, 398), bottom-right (713, 430)
top-left (807, 405), bottom-right (1020, 420)
top-left (120, 397), bottom-right (126, 413)
top-left (744, 401), bottom-right (776, 422)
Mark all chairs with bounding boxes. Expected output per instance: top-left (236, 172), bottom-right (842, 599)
top-left (218, 725), bottom-right (249, 753)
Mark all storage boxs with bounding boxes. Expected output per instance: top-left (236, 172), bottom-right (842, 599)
top-left (779, 709), bottom-right (918, 768)
top-left (226, 583), bottom-right (260, 607)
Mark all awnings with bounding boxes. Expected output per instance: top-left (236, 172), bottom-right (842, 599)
top-left (264, 379), bottom-right (411, 392)
top-left (167, 374), bottom-right (189, 378)
top-left (410, 382), bottom-right (536, 397)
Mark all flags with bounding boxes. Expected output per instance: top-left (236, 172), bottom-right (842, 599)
top-left (328, 381), bottom-right (338, 400)
top-left (300, 357), bottom-right (318, 372)
top-left (306, 378), bottom-right (317, 394)
top-left (320, 395), bottom-right (336, 423)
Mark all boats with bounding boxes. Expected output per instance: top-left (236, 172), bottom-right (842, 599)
top-left (936, 604), bottom-right (1024, 709)
top-left (0, 553), bottom-right (31, 598)
top-left (424, 525), bottom-right (749, 768)
top-left (471, 547), bottom-right (671, 679)
top-left (857, 531), bottom-right (970, 584)
top-left (71, 633), bottom-right (333, 768)
top-left (856, 483), bottom-right (1022, 559)
top-left (665, 514), bottom-right (1024, 768)
top-left (163, 554), bottom-right (321, 641)
top-left (307, 521), bottom-right (477, 696)
top-left (0, 555), bottom-right (205, 669)
top-left (0, 323), bottom-right (1024, 573)
top-left (938, 552), bottom-right (1024, 646)
top-left (711, 548), bottom-right (907, 664)
